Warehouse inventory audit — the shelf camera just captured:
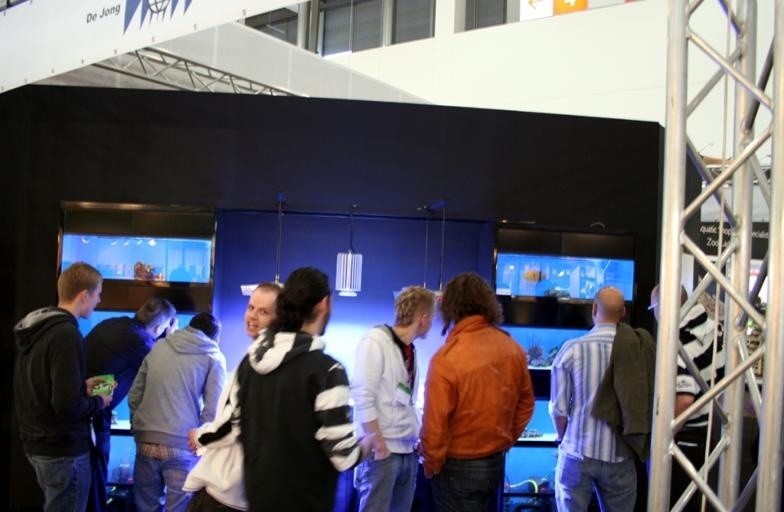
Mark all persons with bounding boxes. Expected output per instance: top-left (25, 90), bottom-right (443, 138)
top-left (647, 280), bottom-right (726, 511)
top-left (418, 270), bottom-right (535, 511)
top-left (234, 264), bottom-right (374, 511)
top-left (545, 283), bottom-right (650, 511)
top-left (181, 280), bottom-right (281, 511)
top-left (83, 296), bottom-right (178, 511)
top-left (126, 311), bottom-right (225, 511)
top-left (352, 283), bottom-right (438, 511)
top-left (11, 260), bottom-right (117, 512)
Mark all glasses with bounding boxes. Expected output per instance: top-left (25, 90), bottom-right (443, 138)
top-left (647, 303), bottom-right (657, 311)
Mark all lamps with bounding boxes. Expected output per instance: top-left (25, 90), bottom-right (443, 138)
top-left (240, 201), bottom-right (288, 299)
top-left (332, 209), bottom-right (363, 301)
top-left (392, 200), bottom-right (448, 313)
